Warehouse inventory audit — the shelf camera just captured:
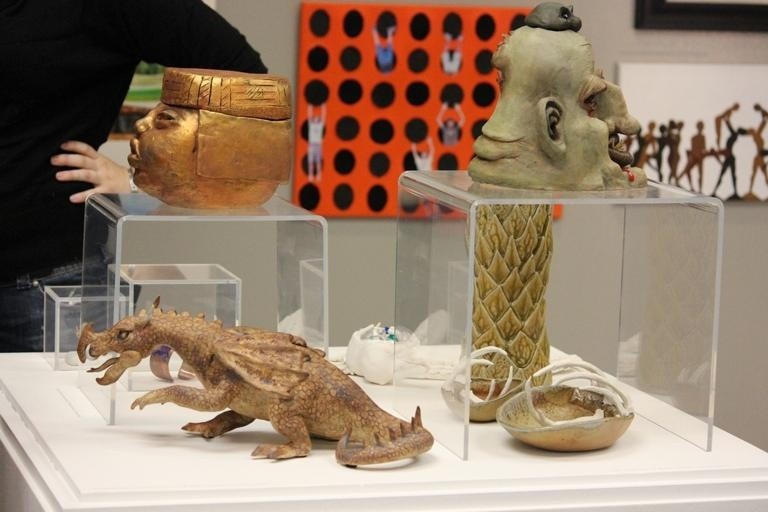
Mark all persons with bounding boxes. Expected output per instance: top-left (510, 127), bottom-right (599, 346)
top-left (370, 26), bottom-right (398, 73)
top-left (0, 1), bottom-right (270, 353)
top-left (305, 101), bottom-right (328, 182)
top-left (408, 136), bottom-right (442, 218)
top-left (435, 100), bottom-right (466, 145)
top-left (127, 64), bottom-right (294, 209)
top-left (439, 31), bottom-right (464, 75)
